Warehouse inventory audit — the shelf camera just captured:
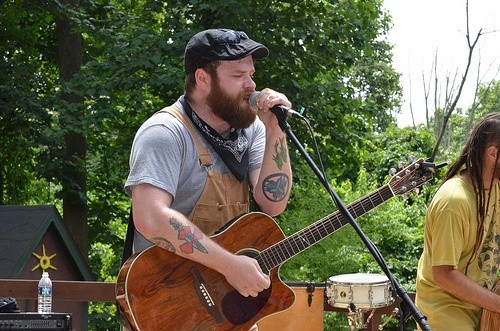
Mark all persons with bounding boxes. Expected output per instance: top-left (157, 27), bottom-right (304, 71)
top-left (124, 28), bottom-right (293, 331)
top-left (414, 112), bottom-right (500, 331)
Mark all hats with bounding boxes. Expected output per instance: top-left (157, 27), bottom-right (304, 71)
top-left (184, 28), bottom-right (270, 74)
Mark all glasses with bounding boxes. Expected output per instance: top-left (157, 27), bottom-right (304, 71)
top-left (211, 30), bottom-right (249, 53)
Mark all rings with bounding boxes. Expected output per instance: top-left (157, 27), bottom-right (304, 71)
top-left (276, 94), bottom-right (279, 98)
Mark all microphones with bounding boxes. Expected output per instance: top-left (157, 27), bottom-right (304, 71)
top-left (249, 91), bottom-right (305, 119)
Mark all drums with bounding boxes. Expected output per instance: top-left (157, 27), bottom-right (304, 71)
top-left (325, 271), bottom-right (395, 311)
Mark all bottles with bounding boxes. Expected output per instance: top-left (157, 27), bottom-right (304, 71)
top-left (38, 272), bottom-right (53, 313)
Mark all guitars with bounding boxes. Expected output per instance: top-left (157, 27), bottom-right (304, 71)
top-left (115, 157), bottom-right (436, 331)
